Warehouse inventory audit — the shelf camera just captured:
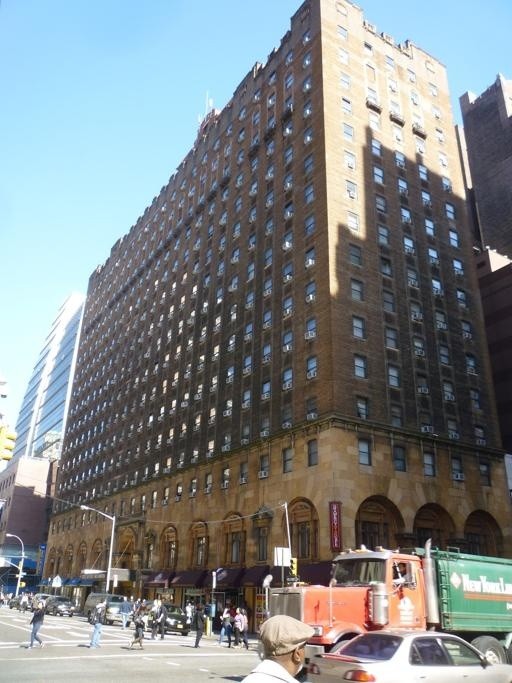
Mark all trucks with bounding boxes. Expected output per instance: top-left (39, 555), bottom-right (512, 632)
top-left (268, 537), bottom-right (512, 665)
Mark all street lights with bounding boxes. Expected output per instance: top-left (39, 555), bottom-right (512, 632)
top-left (279, 498), bottom-right (292, 582)
top-left (6, 533), bottom-right (26, 599)
top-left (211, 566), bottom-right (224, 637)
top-left (262, 574), bottom-right (273, 619)
top-left (80, 505), bottom-right (116, 594)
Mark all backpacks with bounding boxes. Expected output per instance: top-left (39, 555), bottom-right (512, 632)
top-left (88, 607), bottom-right (103, 625)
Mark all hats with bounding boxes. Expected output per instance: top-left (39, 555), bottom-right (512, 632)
top-left (260, 615), bottom-right (315, 656)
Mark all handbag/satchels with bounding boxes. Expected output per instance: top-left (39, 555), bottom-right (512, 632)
top-left (134, 634), bottom-right (144, 639)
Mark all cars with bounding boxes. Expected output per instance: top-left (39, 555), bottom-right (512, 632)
top-left (306, 629), bottom-right (512, 683)
top-left (9, 589), bottom-right (76, 616)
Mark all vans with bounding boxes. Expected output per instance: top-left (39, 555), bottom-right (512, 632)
top-left (83, 591), bottom-right (134, 626)
top-left (134, 599), bottom-right (191, 636)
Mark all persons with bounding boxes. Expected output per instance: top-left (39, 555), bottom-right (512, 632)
top-left (239, 614), bottom-right (316, 682)
top-left (394, 562), bottom-right (407, 586)
top-left (0, 590), bottom-right (250, 650)
